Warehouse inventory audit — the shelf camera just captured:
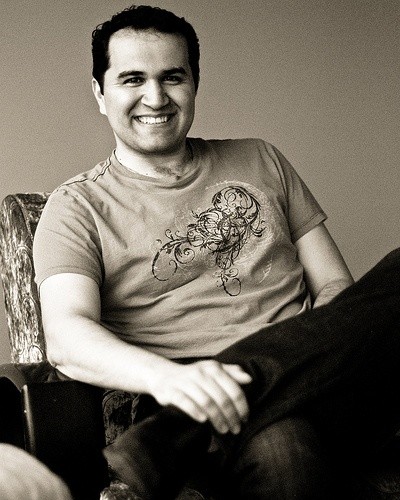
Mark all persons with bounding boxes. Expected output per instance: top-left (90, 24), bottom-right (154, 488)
top-left (33, 5), bottom-right (398, 499)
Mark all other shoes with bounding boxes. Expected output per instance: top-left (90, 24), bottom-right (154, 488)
top-left (103, 409), bottom-right (209, 499)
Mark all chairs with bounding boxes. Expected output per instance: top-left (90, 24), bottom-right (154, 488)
top-left (0, 191), bottom-right (201, 500)
